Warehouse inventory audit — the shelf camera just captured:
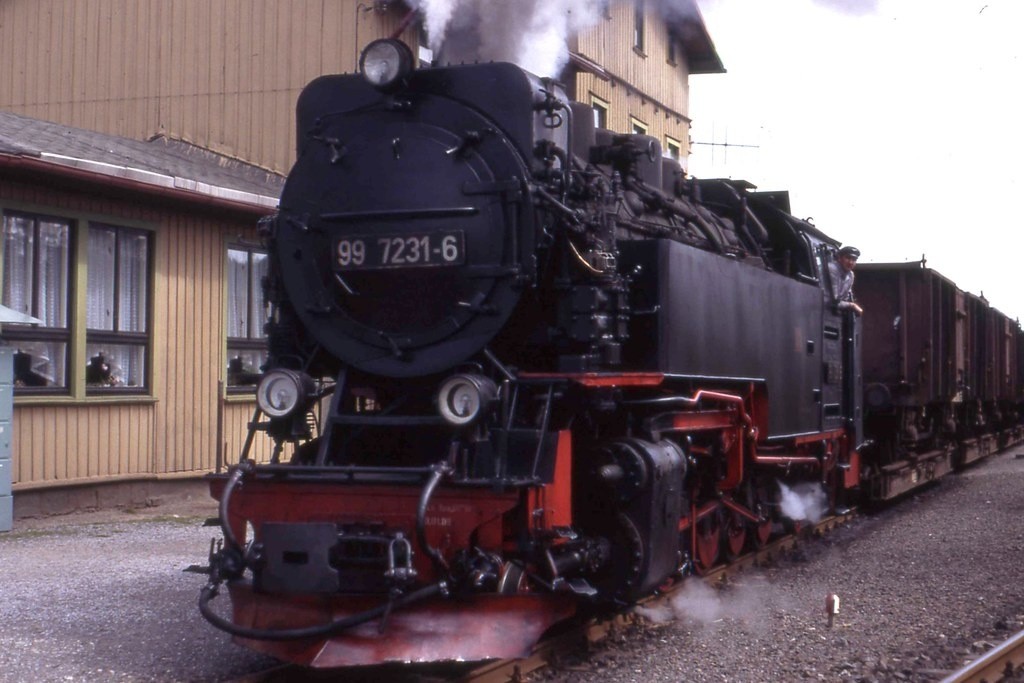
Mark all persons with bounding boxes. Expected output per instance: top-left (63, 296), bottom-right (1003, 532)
top-left (827, 246), bottom-right (863, 318)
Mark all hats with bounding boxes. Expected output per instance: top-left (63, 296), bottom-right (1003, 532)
top-left (841, 247), bottom-right (860, 259)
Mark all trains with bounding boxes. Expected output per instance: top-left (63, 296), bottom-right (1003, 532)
top-left (181, 37), bottom-right (1024, 672)
